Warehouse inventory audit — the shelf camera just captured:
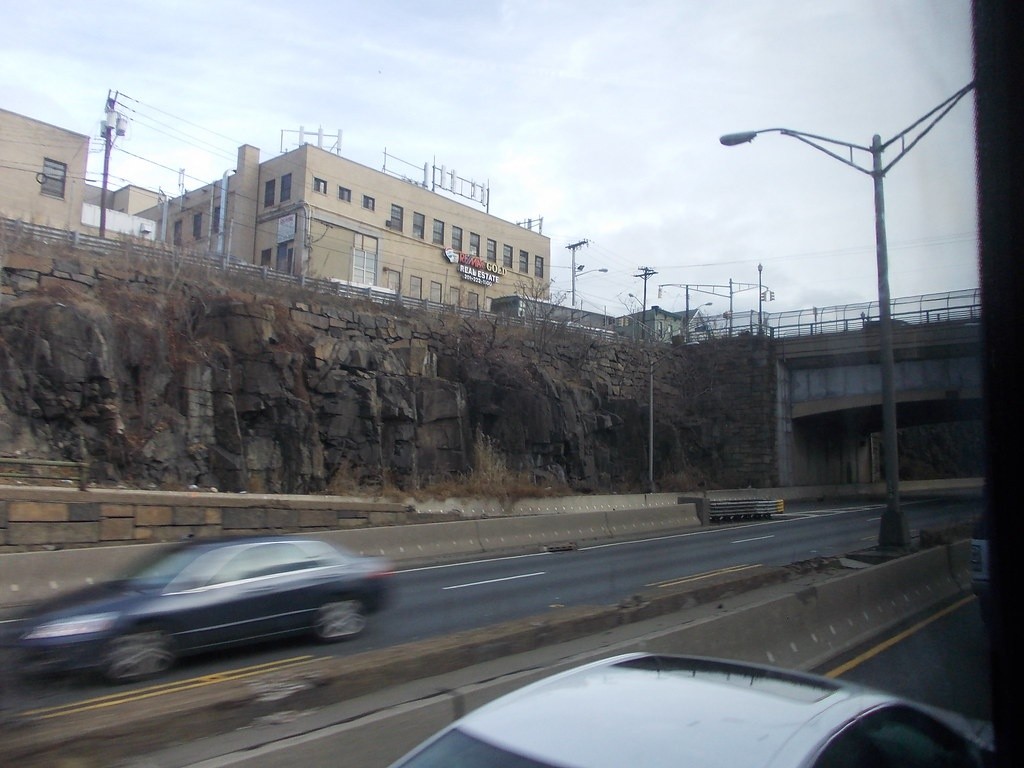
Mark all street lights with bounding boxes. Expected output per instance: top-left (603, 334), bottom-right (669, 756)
top-left (628, 264), bottom-right (658, 341)
top-left (684, 284), bottom-right (715, 344)
top-left (563, 239), bottom-right (608, 310)
top-left (754, 265), bottom-right (767, 335)
top-left (720, 81), bottom-right (978, 551)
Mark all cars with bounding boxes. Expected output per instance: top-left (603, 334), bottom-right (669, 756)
top-left (388, 650), bottom-right (994, 768)
top-left (16, 535), bottom-right (405, 692)
